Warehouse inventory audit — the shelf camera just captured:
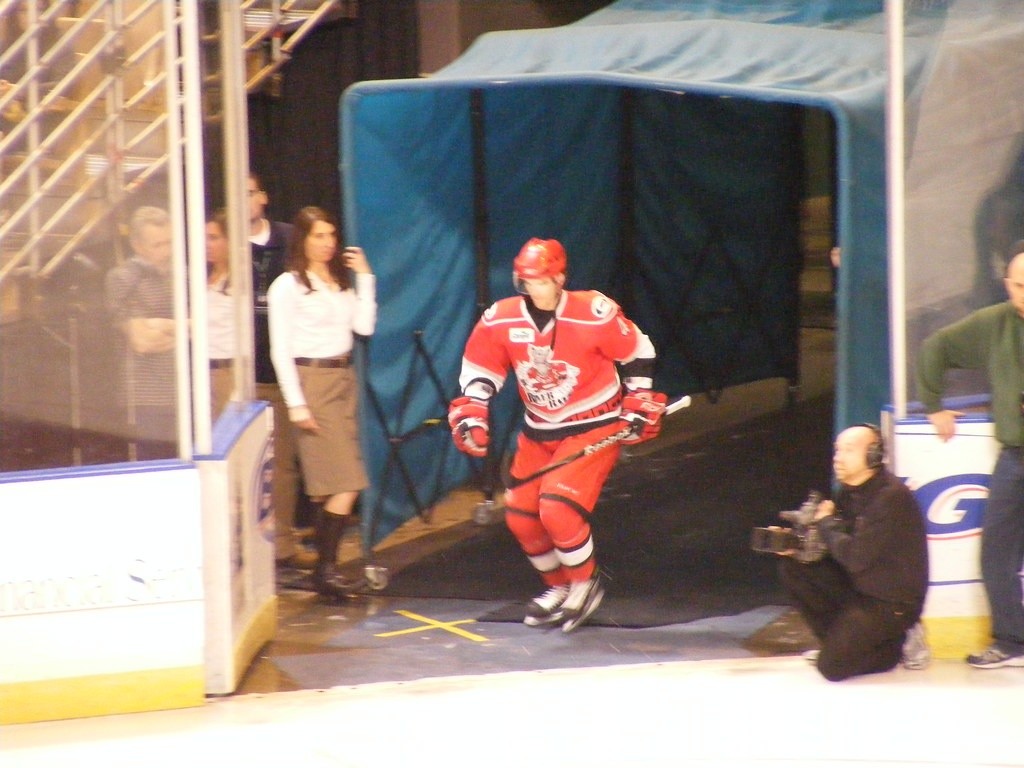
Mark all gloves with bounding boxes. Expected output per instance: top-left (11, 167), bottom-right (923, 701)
top-left (448, 396), bottom-right (491, 457)
top-left (621, 388), bottom-right (668, 444)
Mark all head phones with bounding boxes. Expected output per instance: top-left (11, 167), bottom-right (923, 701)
top-left (854, 421), bottom-right (883, 467)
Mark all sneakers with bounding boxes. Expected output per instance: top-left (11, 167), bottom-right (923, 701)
top-left (967, 646), bottom-right (1024, 669)
top-left (561, 575), bottom-right (605, 632)
top-left (899, 622), bottom-right (930, 670)
top-left (522, 585), bottom-right (570, 629)
top-left (804, 648), bottom-right (821, 665)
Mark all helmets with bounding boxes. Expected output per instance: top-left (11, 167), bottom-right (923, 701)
top-left (513, 237), bottom-right (567, 278)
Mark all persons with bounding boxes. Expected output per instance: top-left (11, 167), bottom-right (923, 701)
top-left (915, 252), bottom-right (1024, 668)
top-left (247, 167), bottom-right (319, 569)
top-left (205, 209), bottom-right (234, 430)
top-left (267, 207), bottom-right (377, 607)
top-left (101, 205), bottom-right (192, 461)
top-left (448, 237), bottom-right (667, 619)
top-left (0, 0), bottom-right (75, 152)
top-left (767, 427), bottom-right (929, 682)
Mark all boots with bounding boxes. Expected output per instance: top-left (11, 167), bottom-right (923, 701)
top-left (307, 500), bottom-right (368, 596)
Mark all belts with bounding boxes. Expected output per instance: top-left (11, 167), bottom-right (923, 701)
top-left (295, 357), bottom-right (352, 368)
top-left (210, 358), bottom-right (234, 369)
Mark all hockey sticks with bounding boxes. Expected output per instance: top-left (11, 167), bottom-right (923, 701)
top-left (481, 392), bottom-right (693, 509)
